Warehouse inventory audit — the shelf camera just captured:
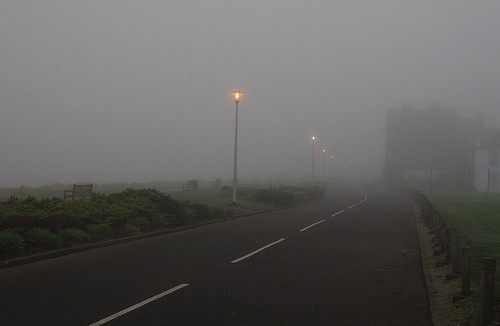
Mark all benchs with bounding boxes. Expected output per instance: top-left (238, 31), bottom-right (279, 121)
top-left (63, 182), bottom-right (94, 200)
top-left (181, 178), bottom-right (200, 191)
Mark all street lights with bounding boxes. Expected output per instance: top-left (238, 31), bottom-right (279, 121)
top-left (312, 135), bottom-right (334, 182)
top-left (230, 89), bottom-right (246, 202)
